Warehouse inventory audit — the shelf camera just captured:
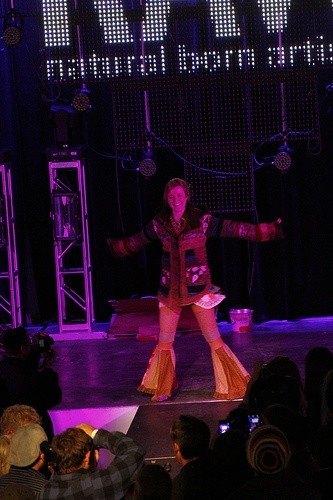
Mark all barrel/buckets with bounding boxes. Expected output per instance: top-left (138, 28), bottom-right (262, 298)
top-left (229, 308), bottom-right (253, 333)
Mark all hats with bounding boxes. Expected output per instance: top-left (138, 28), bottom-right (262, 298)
top-left (9, 423), bottom-right (48, 468)
top-left (246, 424), bottom-right (292, 477)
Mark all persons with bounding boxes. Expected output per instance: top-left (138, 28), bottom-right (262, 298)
top-left (0, 326), bottom-right (332, 500)
top-left (110, 179), bottom-right (283, 402)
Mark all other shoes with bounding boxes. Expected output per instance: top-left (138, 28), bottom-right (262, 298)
top-left (151, 394), bottom-right (168, 402)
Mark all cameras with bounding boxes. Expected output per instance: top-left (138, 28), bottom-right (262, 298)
top-left (218, 420), bottom-right (230, 434)
top-left (248, 414), bottom-right (261, 432)
top-left (33, 336), bottom-right (54, 361)
